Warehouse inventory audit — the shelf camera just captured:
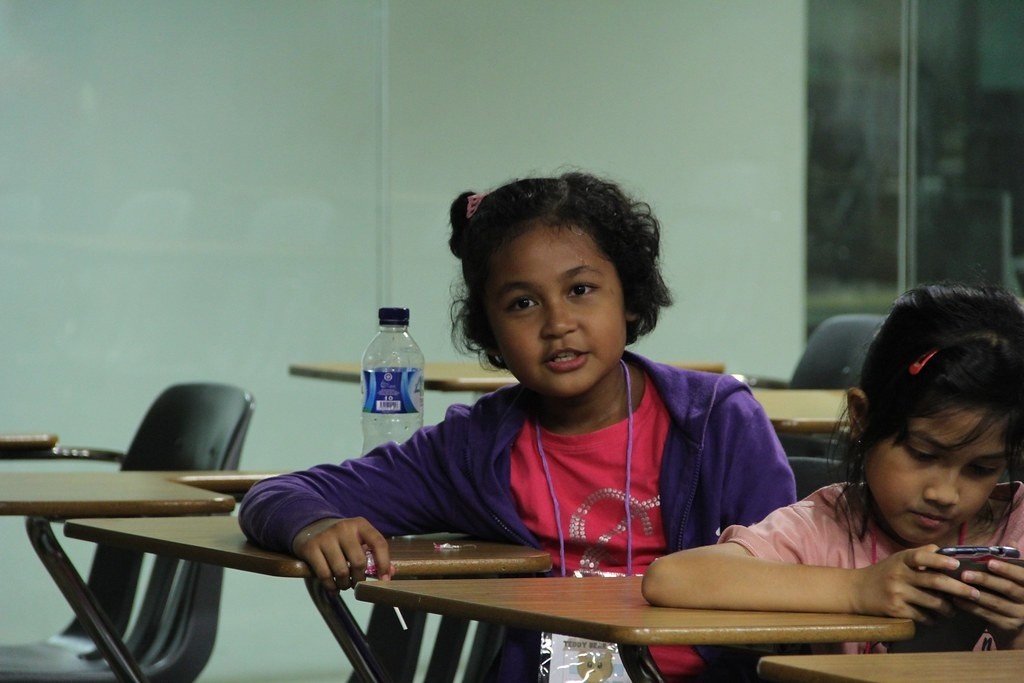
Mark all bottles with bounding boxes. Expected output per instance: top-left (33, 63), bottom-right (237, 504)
top-left (361, 308), bottom-right (425, 457)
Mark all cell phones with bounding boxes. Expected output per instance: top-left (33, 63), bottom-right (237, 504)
top-left (888, 546), bottom-right (1020, 653)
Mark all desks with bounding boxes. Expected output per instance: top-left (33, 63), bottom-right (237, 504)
top-left (0, 360), bottom-right (1024, 683)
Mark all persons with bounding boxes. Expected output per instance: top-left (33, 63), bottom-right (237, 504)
top-left (238, 170), bottom-right (797, 683)
top-left (640, 282), bottom-right (1024, 653)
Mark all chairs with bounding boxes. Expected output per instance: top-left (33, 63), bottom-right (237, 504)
top-left (0, 381), bottom-right (257, 683)
top-left (792, 313), bottom-right (889, 383)
